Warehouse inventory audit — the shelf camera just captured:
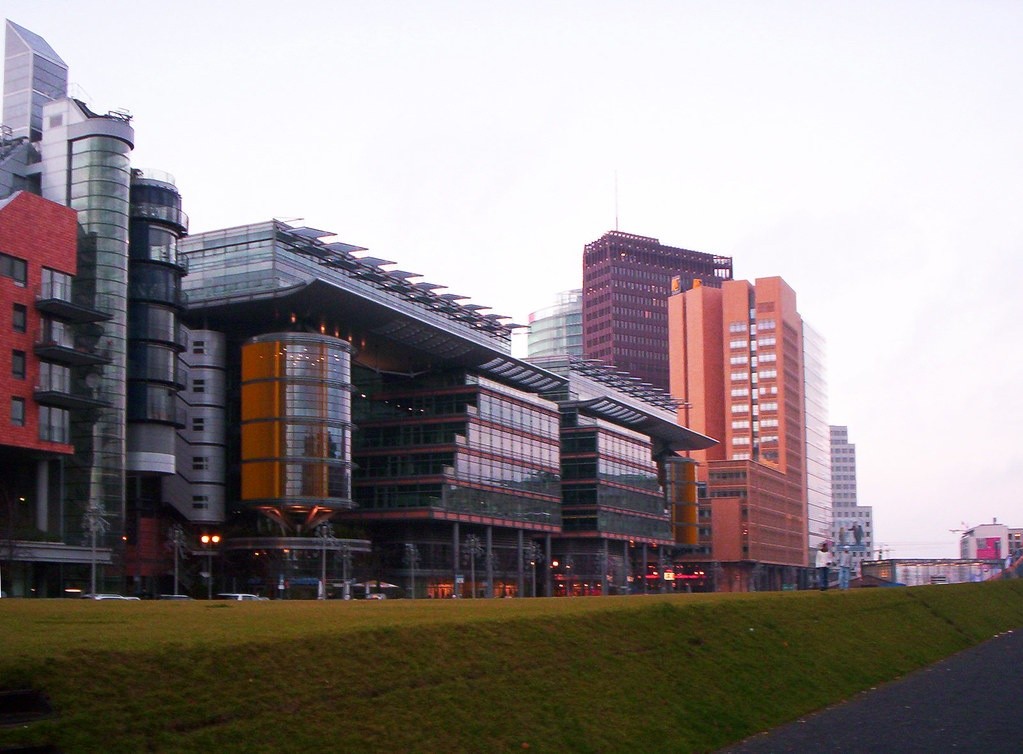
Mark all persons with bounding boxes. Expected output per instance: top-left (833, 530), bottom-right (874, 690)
top-left (283, 576), bottom-right (292, 600)
top-left (1004, 544), bottom-right (1023, 569)
top-left (816, 542), bottom-right (852, 592)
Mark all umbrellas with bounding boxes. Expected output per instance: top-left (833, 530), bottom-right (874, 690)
top-left (355, 580), bottom-right (398, 588)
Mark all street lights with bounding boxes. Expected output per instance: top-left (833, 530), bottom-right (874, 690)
top-left (201, 535), bottom-right (222, 600)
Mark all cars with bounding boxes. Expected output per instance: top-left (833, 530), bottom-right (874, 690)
top-left (216, 593), bottom-right (263, 601)
